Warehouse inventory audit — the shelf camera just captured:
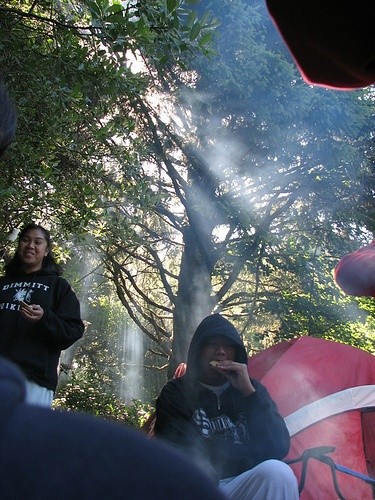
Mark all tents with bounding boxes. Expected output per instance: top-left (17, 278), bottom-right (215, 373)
top-left (141, 335), bottom-right (375, 500)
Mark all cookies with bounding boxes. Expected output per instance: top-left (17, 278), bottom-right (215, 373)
top-left (20, 300), bottom-right (34, 315)
top-left (208, 360), bottom-right (220, 368)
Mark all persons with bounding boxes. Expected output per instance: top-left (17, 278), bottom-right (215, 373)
top-left (0, 223), bottom-right (85, 408)
top-left (156, 313), bottom-right (299, 500)
